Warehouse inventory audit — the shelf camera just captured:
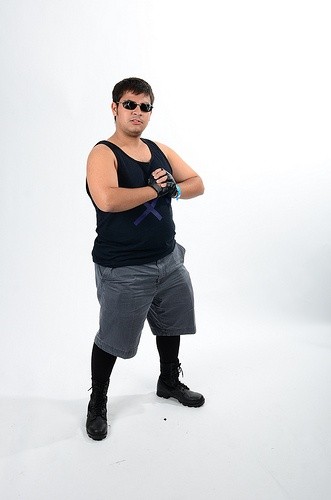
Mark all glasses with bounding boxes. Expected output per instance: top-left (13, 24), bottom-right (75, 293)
top-left (117, 100), bottom-right (153, 112)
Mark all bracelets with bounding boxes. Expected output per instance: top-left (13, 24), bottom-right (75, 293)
top-left (176, 185), bottom-right (182, 200)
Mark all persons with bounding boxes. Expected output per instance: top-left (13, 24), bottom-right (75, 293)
top-left (85, 78), bottom-right (204, 441)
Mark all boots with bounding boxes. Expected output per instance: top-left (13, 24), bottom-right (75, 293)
top-left (86, 378), bottom-right (110, 440)
top-left (157, 360), bottom-right (205, 407)
